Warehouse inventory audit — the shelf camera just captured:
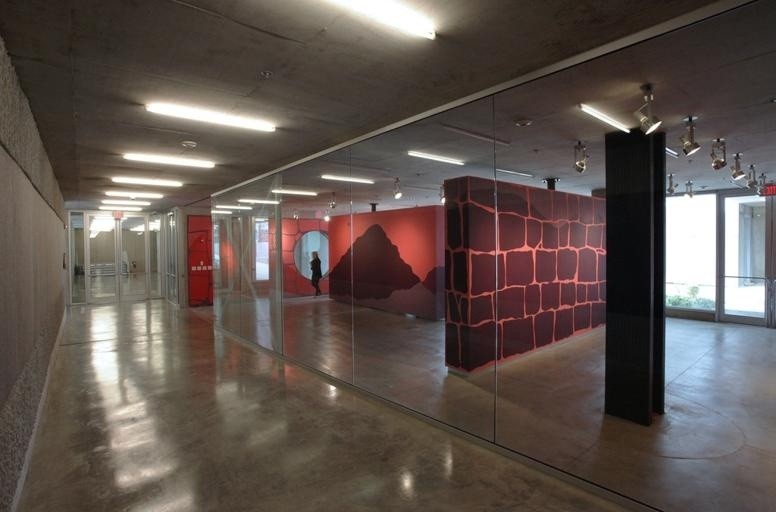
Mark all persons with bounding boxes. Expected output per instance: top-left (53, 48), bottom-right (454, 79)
top-left (310, 250), bottom-right (323, 296)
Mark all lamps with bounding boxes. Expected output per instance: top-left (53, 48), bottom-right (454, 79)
top-left (625, 78), bottom-right (776, 201)
